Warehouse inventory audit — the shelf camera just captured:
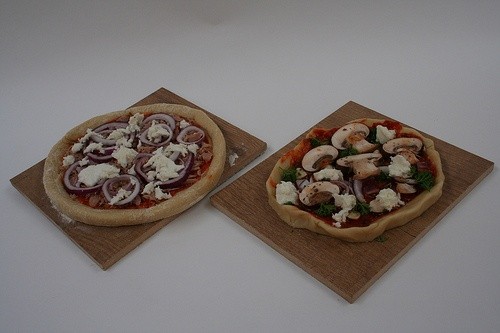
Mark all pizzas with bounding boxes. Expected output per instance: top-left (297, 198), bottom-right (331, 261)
top-left (42, 102), bottom-right (227, 226)
top-left (265, 117), bottom-right (445, 241)
